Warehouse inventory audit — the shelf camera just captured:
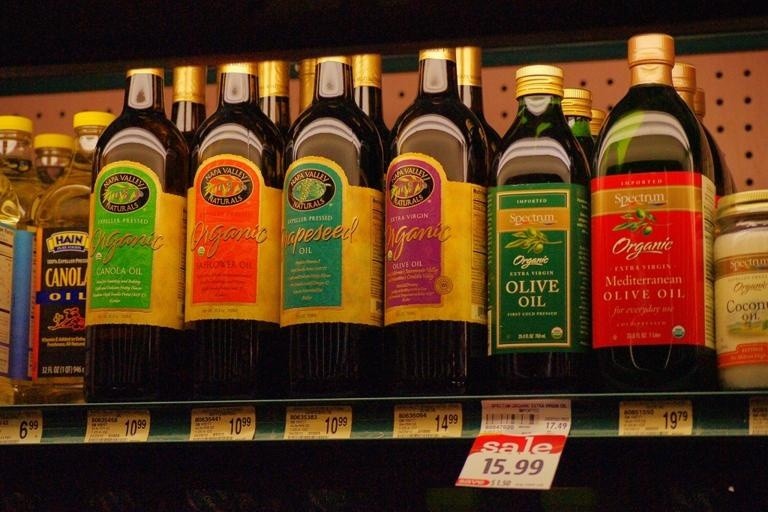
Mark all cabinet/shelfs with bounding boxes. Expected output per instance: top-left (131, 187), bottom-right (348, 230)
top-left (1, 1), bottom-right (764, 459)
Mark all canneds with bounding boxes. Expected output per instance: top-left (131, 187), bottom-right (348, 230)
top-left (713, 189), bottom-right (767, 394)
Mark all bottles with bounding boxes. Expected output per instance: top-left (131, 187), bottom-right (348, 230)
top-left (82, 31), bottom-right (735, 404)
top-left (1, 110), bottom-right (115, 395)
